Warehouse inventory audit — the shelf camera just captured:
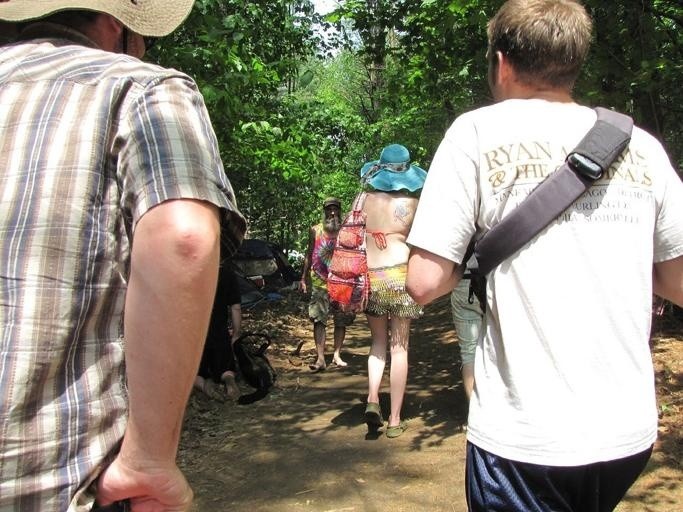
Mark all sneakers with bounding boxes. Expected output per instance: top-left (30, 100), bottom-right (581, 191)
top-left (386, 419), bottom-right (409, 438)
top-left (364, 402), bottom-right (384, 427)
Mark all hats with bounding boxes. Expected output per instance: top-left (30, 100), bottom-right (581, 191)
top-left (360, 144), bottom-right (427, 193)
top-left (323, 197), bottom-right (342, 208)
top-left (0, 0), bottom-right (197, 38)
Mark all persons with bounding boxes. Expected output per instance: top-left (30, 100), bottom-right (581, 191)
top-left (0, 0), bottom-right (244, 509)
top-left (405, 2), bottom-right (683, 512)
top-left (303, 197), bottom-right (356, 369)
top-left (351, 143), bottom-right (429, 439)
top-left (192, 260), bottom-right (243, 403)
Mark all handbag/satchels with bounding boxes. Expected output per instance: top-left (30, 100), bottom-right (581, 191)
top-left (326, 213), bottom-right (371, 314)
top-left (233, 340), bottom-right (277, 389)
